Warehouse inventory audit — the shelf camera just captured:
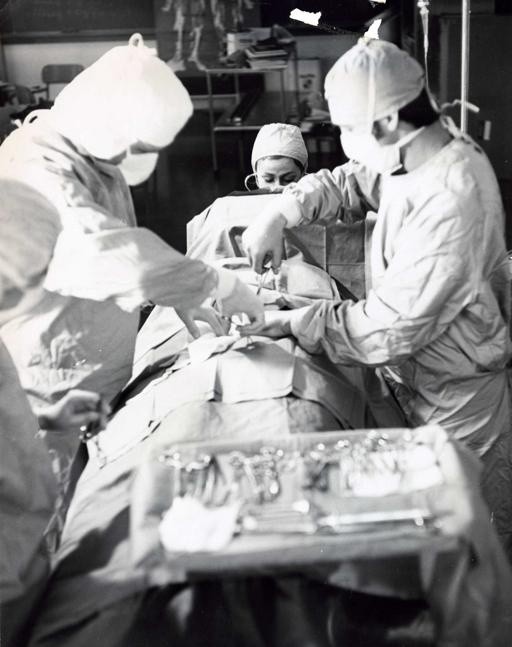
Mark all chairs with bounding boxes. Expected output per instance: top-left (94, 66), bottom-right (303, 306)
top-left (38, 64), bottom-right (86, 101)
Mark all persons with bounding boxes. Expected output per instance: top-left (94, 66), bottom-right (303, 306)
top-left (238, 35), bottom-right (512, 556)
top-left (1, 34), bottom-right (268, 502)
top-left (251, 123), bottom-right (308, 191)
top-left (1, 178), bottom-right (109, 646)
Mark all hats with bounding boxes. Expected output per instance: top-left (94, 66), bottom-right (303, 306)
top-left (252, 122), bottom-right (308, 172)
top-left (47, 32), bottom-right (196, 161)
top-left (324, 38), bottom-right (426, 128)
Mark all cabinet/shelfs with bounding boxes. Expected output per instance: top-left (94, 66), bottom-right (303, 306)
top-left (201, 39), bottom-right (304, 181)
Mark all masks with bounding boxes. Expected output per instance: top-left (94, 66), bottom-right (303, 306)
top-left (340, 128), bottom-right (399, 177)
top-left (116, 148), bottom-right (160, 188)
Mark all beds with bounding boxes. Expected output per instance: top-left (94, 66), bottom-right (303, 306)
top-left (32, 190), bottom-right (430, 644)
